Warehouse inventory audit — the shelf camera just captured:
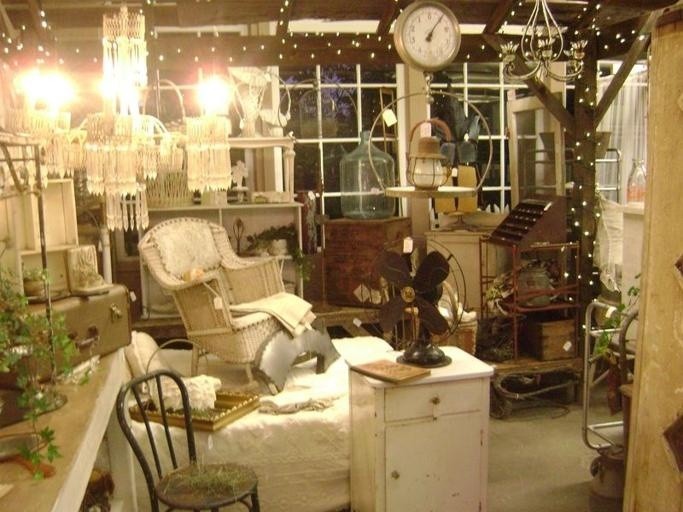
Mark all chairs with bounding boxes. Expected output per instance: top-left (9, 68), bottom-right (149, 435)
top-left (138, 217), bottom-right (340, 395)
top-left (118, 370), bottom-right (261, 511)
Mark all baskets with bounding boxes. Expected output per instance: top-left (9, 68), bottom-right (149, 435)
top-left (232, 82), bottom-right (265, 139)
top-left (589, 444), bottom-right (626, 508)
top-left (249, 71), bottom-right (292, 137)
top-left (430, 320), bottom-right (478, 357)
top-left (138, 80), bottom-right (194, 208)
top-left (146, 340), bottom-right (221, 413)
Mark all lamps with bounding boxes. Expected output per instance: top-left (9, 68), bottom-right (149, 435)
top-left (0, 9), bottom-right (232, 230)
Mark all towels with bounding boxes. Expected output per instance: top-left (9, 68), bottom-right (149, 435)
top-left (229, 293), bottom-right (317, 338)
top-left (260, 386), bottom-right (342, 415)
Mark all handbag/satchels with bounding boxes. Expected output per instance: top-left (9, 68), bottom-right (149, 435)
top-left (595, 263), bottom-right (620, 328)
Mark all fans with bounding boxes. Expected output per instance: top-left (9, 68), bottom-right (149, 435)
top-left (366, 235), bottom-right (467, 368)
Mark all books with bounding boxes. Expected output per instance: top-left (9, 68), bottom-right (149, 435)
top-left (349, 358), bottom-right (432, 387)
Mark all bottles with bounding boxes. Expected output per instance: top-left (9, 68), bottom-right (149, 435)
top-left (626, 159), bottom-right (648, 210)
top-left (517, 267), bottom-right (550, 307)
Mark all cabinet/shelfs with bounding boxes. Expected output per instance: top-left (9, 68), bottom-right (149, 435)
top-left (139, 137), bottom-right (303, 321)
top-left (347, 344), bottom-right (493, 511)
top-left (0, 180), bottom-right (80, 308)
top-left (322, 216), bottom-right (412, 308)
top-left (477, 237), bottom-right (579, 417)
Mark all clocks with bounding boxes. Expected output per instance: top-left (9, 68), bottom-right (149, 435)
top-left (394, 2), bottom-right (462, 74)
